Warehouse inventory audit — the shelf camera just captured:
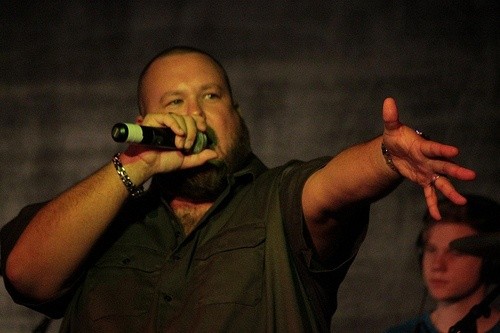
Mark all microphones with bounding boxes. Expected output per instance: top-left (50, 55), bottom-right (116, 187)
top-left (111, 122), bottom-right (207, 153)
